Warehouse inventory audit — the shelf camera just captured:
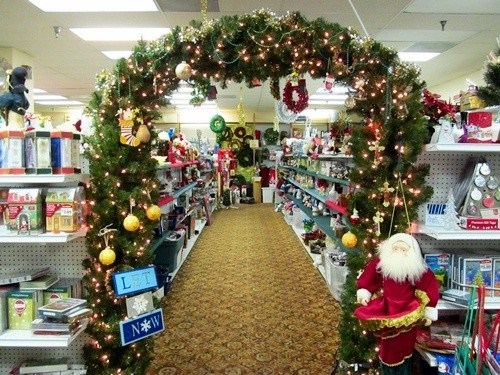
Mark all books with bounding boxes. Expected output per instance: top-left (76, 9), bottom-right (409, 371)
top-left (0, 266), bottom-right (93, 375)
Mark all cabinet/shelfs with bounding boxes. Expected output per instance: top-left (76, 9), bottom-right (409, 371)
top-left (281, 155), bottom-right (355, 281)
top-left (149, 160), bottom-right (218, 282)
top-left (416, 142), bottom-right (500, 308)
top-left (0, 174), bottom-right (89, 375)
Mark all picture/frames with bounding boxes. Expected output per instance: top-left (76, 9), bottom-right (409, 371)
top-left (424, 254), bottom-right (500, 298)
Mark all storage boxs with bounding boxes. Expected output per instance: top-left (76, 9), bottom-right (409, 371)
top-left (324, 252), bottom-right (349, 287)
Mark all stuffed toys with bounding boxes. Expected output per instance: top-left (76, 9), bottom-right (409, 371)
top-left (356, 233), bottom-right (439, 375)
